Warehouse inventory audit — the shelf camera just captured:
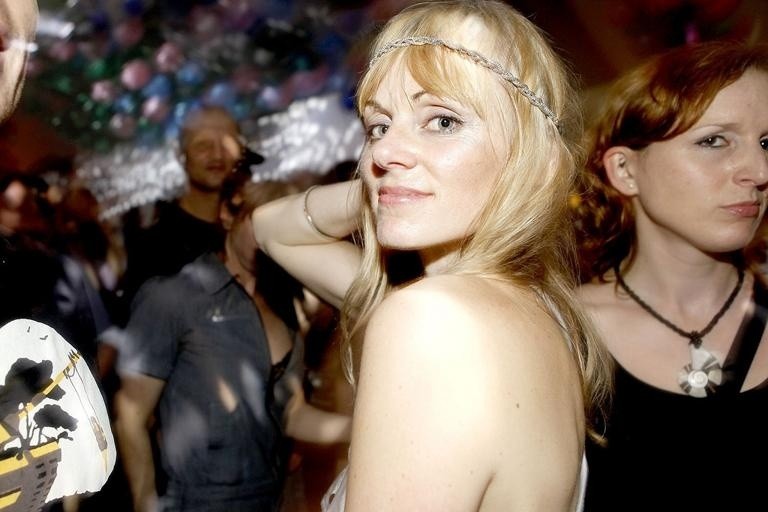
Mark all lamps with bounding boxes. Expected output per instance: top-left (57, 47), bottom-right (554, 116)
top-left (0, 319), bottom-right (116, 511)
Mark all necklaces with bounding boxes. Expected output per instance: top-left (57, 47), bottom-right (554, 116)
top-left (613, 256), bottom-right (749, 400)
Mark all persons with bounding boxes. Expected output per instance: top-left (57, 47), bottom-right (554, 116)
top-left (30, 1), bottom-right (360, 144)
top-left (554, 32), bottom-right (767, 511)
top-left (2, 102), bottom-right (429, 511)
top-left (249, 0), bottom-right (620, 512)
